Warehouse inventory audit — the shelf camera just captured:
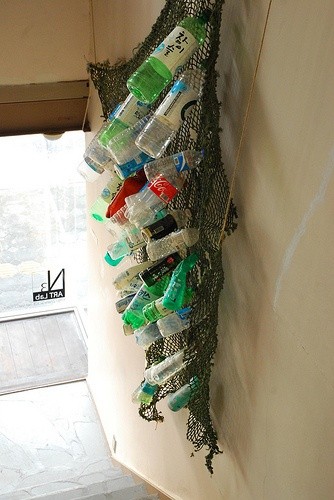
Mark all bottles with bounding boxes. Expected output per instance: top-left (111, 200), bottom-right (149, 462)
top-left (82, 10), bottom-right (213, 411)
